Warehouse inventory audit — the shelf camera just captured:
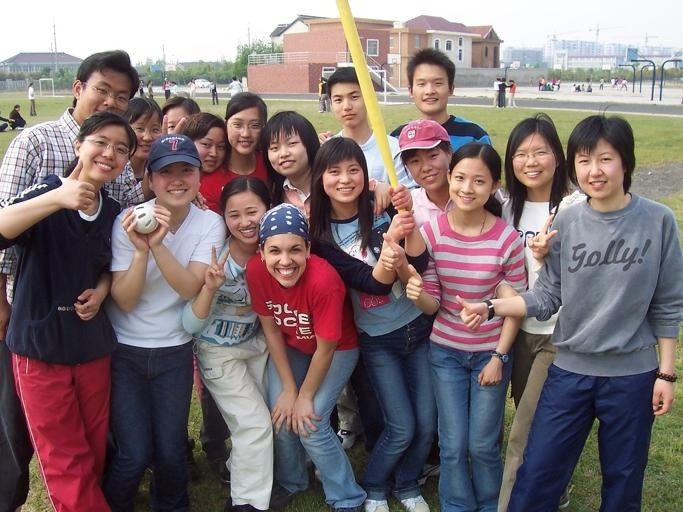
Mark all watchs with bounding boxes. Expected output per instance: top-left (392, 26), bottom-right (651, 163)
top-left (491, 350), bottom-right (509, 364)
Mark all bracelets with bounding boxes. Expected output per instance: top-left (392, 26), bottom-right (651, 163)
top-left (484, 299), bottom-right (496, 321)
top-left (655, 371), bottom-right (678, 382)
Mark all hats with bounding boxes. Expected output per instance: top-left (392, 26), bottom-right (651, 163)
top-left (394, 118), bottom-right (451, 159)
top-left (148, 133), bottom-right (202, 171)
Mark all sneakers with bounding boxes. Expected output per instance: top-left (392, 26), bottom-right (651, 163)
top-left (208, 455), bottom-right (230, 484)
top-left (269, 484), bottom-right (297, 509)
top-left (336, 494), bottom-right (430, 512)
top-left (186, 439), bottom-right (202, 483)
top-left (337, 429), bottom-right (364, 450)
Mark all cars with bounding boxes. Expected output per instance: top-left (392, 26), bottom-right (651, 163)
top-left (187, 78), bottom-right (211, 88)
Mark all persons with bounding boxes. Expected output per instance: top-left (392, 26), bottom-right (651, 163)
top-left (493, 78), bottom-right (517, 109)
top-left (104, 133), bottom-right (228, 512)
top-left (404, 141), bottom-right (527, 512)
top-left (312, 51), bottom-right (492, 450)
top-left (318, 76), bottom-right (331, 113)
top-left (455, 114), bottom-right (680, 512)
top-left (1, 49), bottom-right (186, 512)
top-left (308, 139), bottom-right (435, 511)
top-left (179, 176), bottom-right (278, 512)
top-left (496, 113), bottom-right (571, 508)
top-left (8, 104), bottom-right (27, 131)
top-left (1, 117), bottom-right (15, 132)
top-left (124, 94), bottom-right (357, 481)
top-left (538, 77), bottom-right (628, 93)
top-left (246, 202), bottom-right (366, 512)
top-left (139, 76), bottom-right (243, 105)
top-left (2, 108), bottom-right (138, 512)
top-left (28, 82), bottom-right (38, 117)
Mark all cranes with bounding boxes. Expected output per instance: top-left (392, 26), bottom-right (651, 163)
top-left (588, 23), bottom-right (609, 43)
top-left (643, 32), bottom-right (658, 46)
top-left (545, 30), bottom-right (564, 40)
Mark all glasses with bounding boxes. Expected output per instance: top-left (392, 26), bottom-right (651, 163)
top-left (83, 82), bottom-right (132, 105)
top-left (512, 151), bottom-right (553, 160)
top-left (84, 138), bottom-right (130, 157)
